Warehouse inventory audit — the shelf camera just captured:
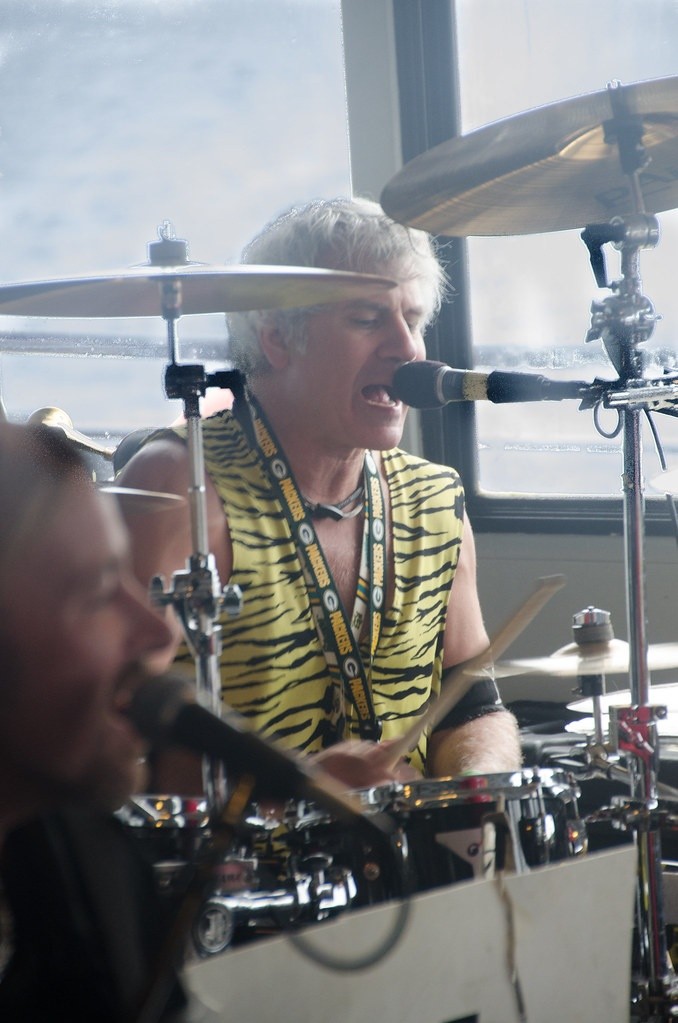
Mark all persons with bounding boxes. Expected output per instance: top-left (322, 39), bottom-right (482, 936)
top-left (0, 417), bottom-right (211, 1023)
top-left (110, 200), bottom-right (522, 880)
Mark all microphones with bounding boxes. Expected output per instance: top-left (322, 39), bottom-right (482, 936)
top-left (391, 356), bottom-right (586, 409)
top-left (124, 672), bottom-right (395, 852)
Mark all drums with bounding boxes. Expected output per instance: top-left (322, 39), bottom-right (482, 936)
top-left (285, 766), bottom-right (569, 909)
top-left (109, 795), bottom-right (267, 904)
top-left (563, 683), bottom-right (677, 741)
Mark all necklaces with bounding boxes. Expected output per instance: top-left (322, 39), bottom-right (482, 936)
top-left (302, 485), bottom-right (365, 520)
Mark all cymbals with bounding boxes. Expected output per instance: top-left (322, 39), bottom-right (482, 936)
top-left (0, 265), bottom-right (400, 318)
top-left (461, 636), bottom-right (677, 676)
top-left (380, 76), bottom-right (678, 237)
